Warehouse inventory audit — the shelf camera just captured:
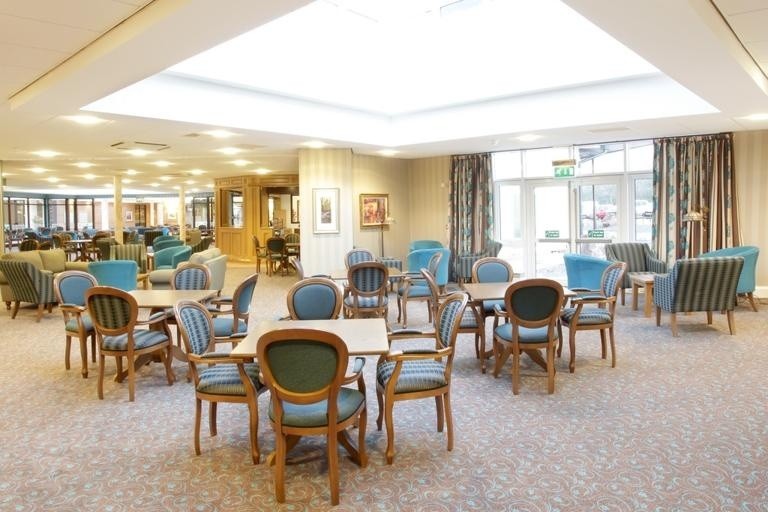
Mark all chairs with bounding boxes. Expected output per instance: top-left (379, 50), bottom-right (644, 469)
top-left (251, 234), bottom-right (273, 274)
top-left (170, 264), bottom-right (211, 348)
top-left (472, 256), bottom-right (513, 332)
top-left (84, 286), bottom-right (174, 401)
top-left (284, 234), bottom-right (300, 259)
top-left (267, 237), bottom-right (287, 278)
top-left (493, 280), bottom-right (565, 395)
top-left (286, 278), bottom-right (367, 428)
top-left (291, 258), bottom-right (334, 282)
top-left (421, 267), bottom-right (488, 375)
top-left (555, 261), bottom-right (626, 376)
top-left (344, 248), bottom-right (375, 270)
top-left (53, 270), bottom-right (99, 378)
top-left (343, 262), bottom-right (389, 318)
top-left (256, 327), bottom-right (367, 504)
top-left (174, 299), bottom-right (269, 465)
top-left (38, 240), bottom-right (51, 250)
top-left (20, 239), bottom-right (38, 252)
top-left (376, 293), bottom-right (468, 465)
top-left (185, 273), bottom-right (259, 382)
top-left (397, 252), bottom-right (443, 329)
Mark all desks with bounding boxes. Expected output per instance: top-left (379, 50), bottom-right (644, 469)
top-left (331, 267), bottom-right (405, 280)
top-left (630, 275), bottom-right (692, 318)
top-left (113, 289), bottom-right (218, 385)
top-left (230, 318), bottom-right (389, 465)
top-left (463, 280), bottom-right (577, 376)
top-left (137, 273), bottom-right (150, 289)
top-left (65, 239), bottom-right (93, 261)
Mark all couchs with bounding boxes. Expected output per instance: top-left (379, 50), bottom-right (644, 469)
top-left (150, 249), bottom-right (228, 318)
top-left (154, 240), bottom-right (184, 252)
top-left (1, 259), bottom-right (57, 321)
top-left (564, 253), bottom-right (615, 308)
top-left (455, 243), bottom-right (503, 290)
top-left (154, 246), bottom-right (192, 272)
top-left (406, 248), bottom-right (451, 294)
top-left (377, 257), bottom-right (403, 291)
top-left (88, 260), bottom-right (137, 291)
top-left (700, 245), bottom-right (759, 314)
top-left (410, 241), bottom-right (444, 252)
top-left (153, 235), bottom-right (177, 245)
top-left (110, 243), bottom-right (148, 273)
top-left (0, 249), bottom-right (88, 312)
top-left (605, 243), bottom-right (666, 306)
top-left (192, 236), bottom-right (213, 252)
top-left (654, 256), bottom-right (745, 338)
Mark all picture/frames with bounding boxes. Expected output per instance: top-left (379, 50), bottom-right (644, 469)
top-left (311, 187), bottom-right (340, 234)
top-left (359, 193), bottom-right (389, 227)
